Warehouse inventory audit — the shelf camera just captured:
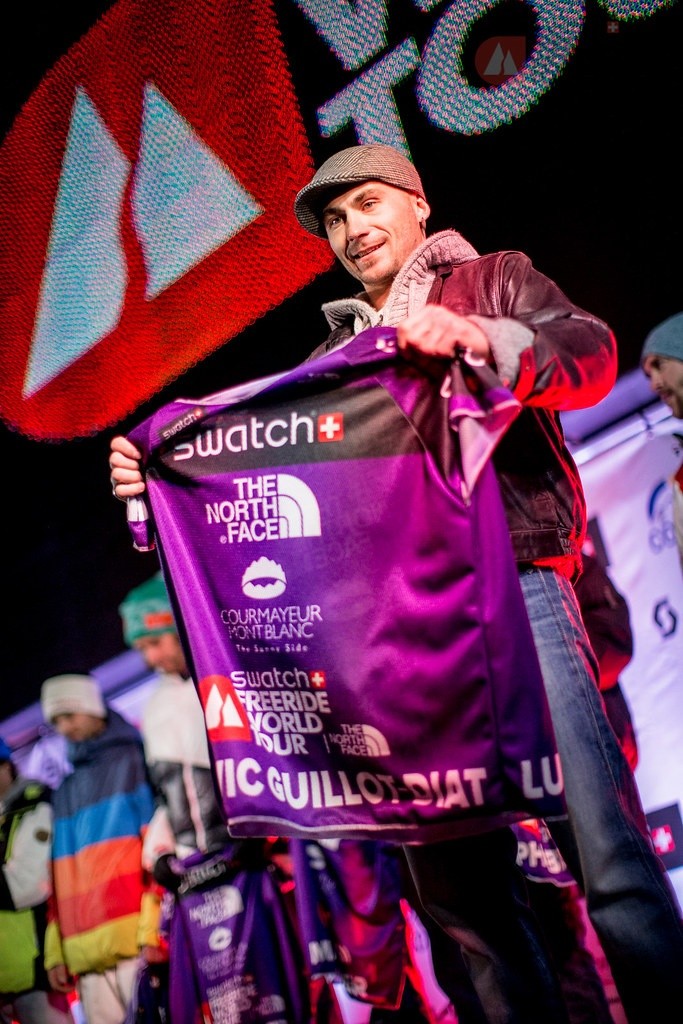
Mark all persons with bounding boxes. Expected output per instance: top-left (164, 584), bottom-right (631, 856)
top-left (543, 552), bottom-right (641, 1004)
top-left (640, 312), bottom-right (679, 428)
top-left (2, 569), bottom-right (340, 1021)
top-left (113, 146), bottom-right (681, 1021)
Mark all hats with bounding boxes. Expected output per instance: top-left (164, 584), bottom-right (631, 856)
top-left (42, 674), bottom-right (104, 724)
top-left (121, 580), bottom-right (178, 642)
top-left (642, 315), bottom-right (682, 361)
top-left (294, 146), bottom-right (426, 238)
